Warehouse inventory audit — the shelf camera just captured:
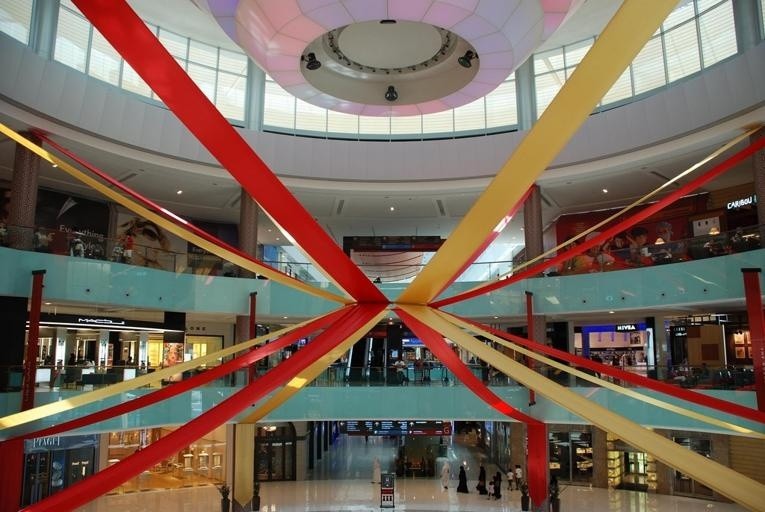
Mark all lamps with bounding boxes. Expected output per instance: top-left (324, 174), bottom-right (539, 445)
top-left (458, 50), bottom-right (478, 68)
top-left (380, 19), bottom-right (396, 24)
top-left (301, 53), bottom-right (321, 70)
top-left (385, 86), bottom-right (398, 101)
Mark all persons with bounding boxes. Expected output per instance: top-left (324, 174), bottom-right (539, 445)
top-left (475, 465), bottom-right (501, 501)
top-left (69, 230), bottom-right (106, 259)
top-left (369, 456), bottom-right (382, 484)
top-left (456, 465), bottom-right (469, 494)
top-left (119, 233), bottom-right (134, 264)
top-left (514, 464), bottom-right (523, 490)
top-left (591, 351), bottom-right (633, 385)
top-left (44, 353), bottom-right (145, 378)
top-left (395, 358), bottom-right (407, 386)
top-left (506, 468), bottom-right (514, 491)
top-left (438, 459), bottom-right (451, 490)
top-left (373, 276), bottom-right (382, 283)
top-left (562, 226), bottom-right (654, 266)
top-left (35, 226), bottom-right (50, 252)
